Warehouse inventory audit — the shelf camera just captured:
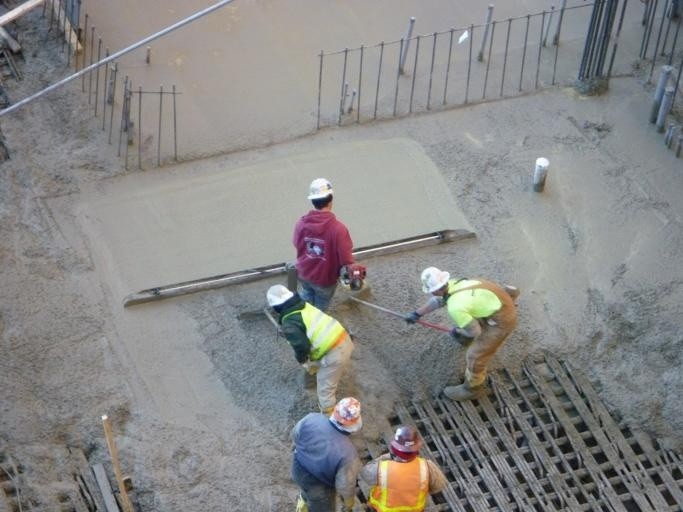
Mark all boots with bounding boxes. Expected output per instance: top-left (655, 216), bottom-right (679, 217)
top-left (444, 369), bottom-right (487, 400)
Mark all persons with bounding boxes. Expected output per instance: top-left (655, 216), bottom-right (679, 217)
top-left (264, 283), bottom-right (355, 417)
top-left (287, 394), bottom-right (364, 511)
top-left (359, 424), bottom-right (446, 511)
top-left (293, 176), bottom-right (353, 305)
top-left (405, 267), bottom-right (517, 400)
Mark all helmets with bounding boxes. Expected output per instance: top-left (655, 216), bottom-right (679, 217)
top-left (329, 397), bottom-right (362, 433)
top-left (421, 267), bottom-right (450, 293)
top-left (267, 284), bottom-right (293, 307)
top-left (390, 425), bottom-right (422, 453)
top-left (308, 178), bottom-right (333, 199)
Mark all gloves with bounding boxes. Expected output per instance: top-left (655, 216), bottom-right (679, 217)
top-left (405, 312), bottom-right (421, 323)
top-left (452, 329), bottom-right (470, 345)
